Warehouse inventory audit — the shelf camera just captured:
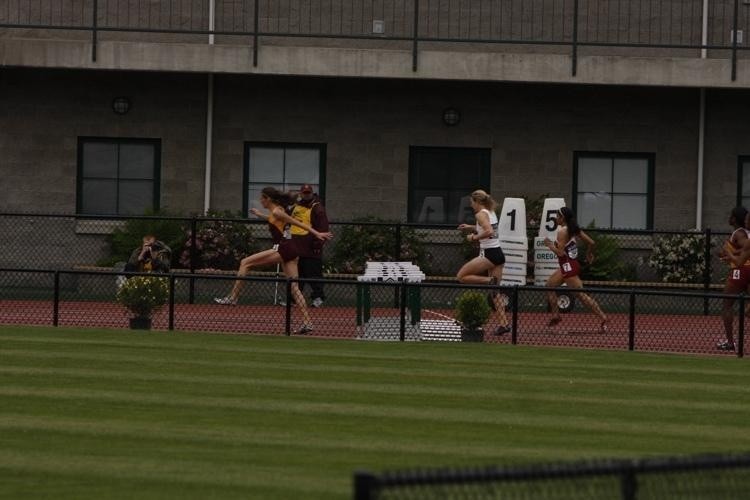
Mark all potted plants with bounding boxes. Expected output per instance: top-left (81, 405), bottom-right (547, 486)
top-left (453, 290), bottom-right (493, 343)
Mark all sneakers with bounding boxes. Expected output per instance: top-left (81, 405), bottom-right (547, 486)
top-left (295, 322), bottom-right (312, 333)
top-left (312, 298), bottom-right (324, 308)
top-left (598, 320), bottom-right (609, 333)
top-left (489, 277), bottom-right (497, 298)
top-left (214, 298), bottom-right (238, 306)
top-left (279, 299), bottom-right (296, 307)
top-left (494, 325), bottom-right (510, 335)
top-left (549, 316), bottom-right (563, 327)
top-left (717, 342), bottom-right (735, 350)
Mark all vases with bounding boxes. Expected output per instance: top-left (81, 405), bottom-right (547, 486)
top-left (129, 318), bottom-right (153, 331)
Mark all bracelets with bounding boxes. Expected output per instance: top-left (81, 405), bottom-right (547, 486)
top-left (471, 234), bottom-right (475, 242)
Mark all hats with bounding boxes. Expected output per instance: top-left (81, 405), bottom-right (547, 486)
top-left (301, 185), bottom-right (313, 193)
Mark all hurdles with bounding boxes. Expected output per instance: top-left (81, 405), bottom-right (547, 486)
top-left (354, 260), bottom-right (462, 343)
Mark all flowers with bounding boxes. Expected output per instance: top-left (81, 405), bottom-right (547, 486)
top-left (116, 272), bottom-right (169, 320)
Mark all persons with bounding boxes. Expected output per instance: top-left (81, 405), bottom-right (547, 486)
top-left (212, 185), bottom-right (335, 335)
top-left (542, 206), bottom-right (609, 335)
top-left (279, 184), bottom-right (330, 307)
top-left (714, 205), bottom-right (750, 351)
top-left (123, 234), bottom-right (180, 306)
top-left (456, 188), bottom-right (511, 336)
top-left (720, 212), bottom-right (750, 267)
